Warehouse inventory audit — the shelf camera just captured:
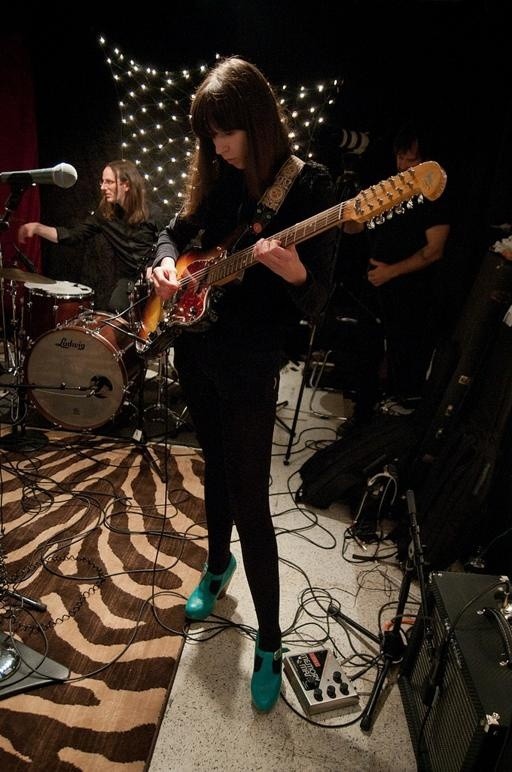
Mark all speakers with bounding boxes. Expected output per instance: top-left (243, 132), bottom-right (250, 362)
top-left (398, 571), bottom-right (512, 771)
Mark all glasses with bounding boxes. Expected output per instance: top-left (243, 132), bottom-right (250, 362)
top-left (98, 179), bottom-right (116, 186)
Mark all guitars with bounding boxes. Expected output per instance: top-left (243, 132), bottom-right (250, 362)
top-left (134, 161), bottom-right (449, 353)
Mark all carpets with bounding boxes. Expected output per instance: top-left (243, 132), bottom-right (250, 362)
top-left (0, 419), bottom-right (211, 771)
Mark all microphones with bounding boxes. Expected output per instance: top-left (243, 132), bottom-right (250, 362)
top-left (8, 243), bottom-right (34, 272)
top-left (36, 158), bottom-right (79, 190)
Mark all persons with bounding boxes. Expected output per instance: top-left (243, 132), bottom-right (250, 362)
top-left (17, 158), bottom-right (159, 366)
top-left (368, 130), bottom-right (452, 414)
top-left (146, 56), bottom-right (345, 715)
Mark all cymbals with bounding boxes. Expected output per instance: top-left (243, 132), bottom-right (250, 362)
top-left (1, 268), bottom-right (56, 284)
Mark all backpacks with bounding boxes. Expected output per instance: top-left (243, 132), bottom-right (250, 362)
top-left (292, 413), bottom-right (422, 507)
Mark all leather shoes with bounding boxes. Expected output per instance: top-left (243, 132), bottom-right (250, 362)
top-left (344, 387), bottom-right (367, 401)
top-left (337, 414), bottom-right (359, 437)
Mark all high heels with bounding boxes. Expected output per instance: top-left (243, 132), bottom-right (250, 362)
top-left (250, 630), bottom-right (282, 714)
top-left (184, 553), bottom-right (237, 622)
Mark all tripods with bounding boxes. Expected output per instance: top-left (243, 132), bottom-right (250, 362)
top-left (322, 569), bottom-right (407, 731)
top-left (96, 370), bottom-right (178, 480)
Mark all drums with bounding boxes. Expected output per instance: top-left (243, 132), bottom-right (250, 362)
top-left (20, 281), bottom-right (95, 355)
top-left (23, 310), bottom-right (151, 431)
